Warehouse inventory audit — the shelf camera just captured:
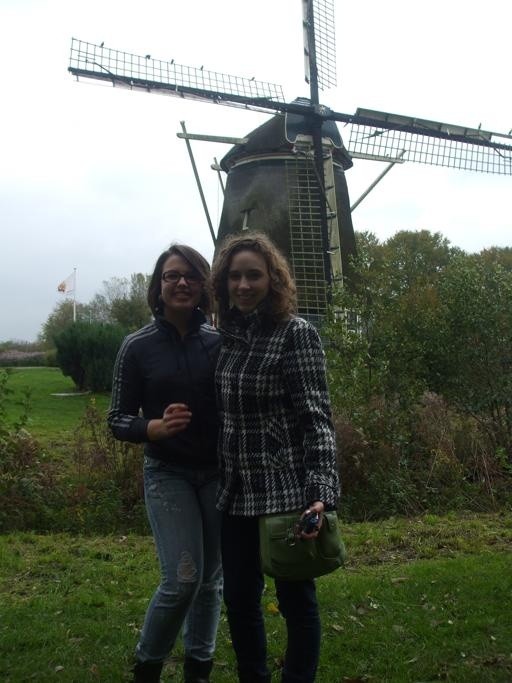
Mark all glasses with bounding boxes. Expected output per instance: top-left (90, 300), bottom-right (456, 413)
top-left (162, 270), bottom-right (202, 285)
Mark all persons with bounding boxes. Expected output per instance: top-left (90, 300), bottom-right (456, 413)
top-left (208, 227), bottom-right (342, 681)
top-left (106, 240), bottom-right (230, 681)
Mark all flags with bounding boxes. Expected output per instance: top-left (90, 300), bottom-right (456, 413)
top-left (57, 270), bottom-right (75, 297)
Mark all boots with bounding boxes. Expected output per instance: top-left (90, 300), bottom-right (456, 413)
top-left (184, 657), bottom-right (213, 683)
top-left (133, 662), bottom-right (163, 683)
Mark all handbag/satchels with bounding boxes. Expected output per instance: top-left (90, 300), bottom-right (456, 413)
top-left (259, 510), bottom-right (346, 580)
